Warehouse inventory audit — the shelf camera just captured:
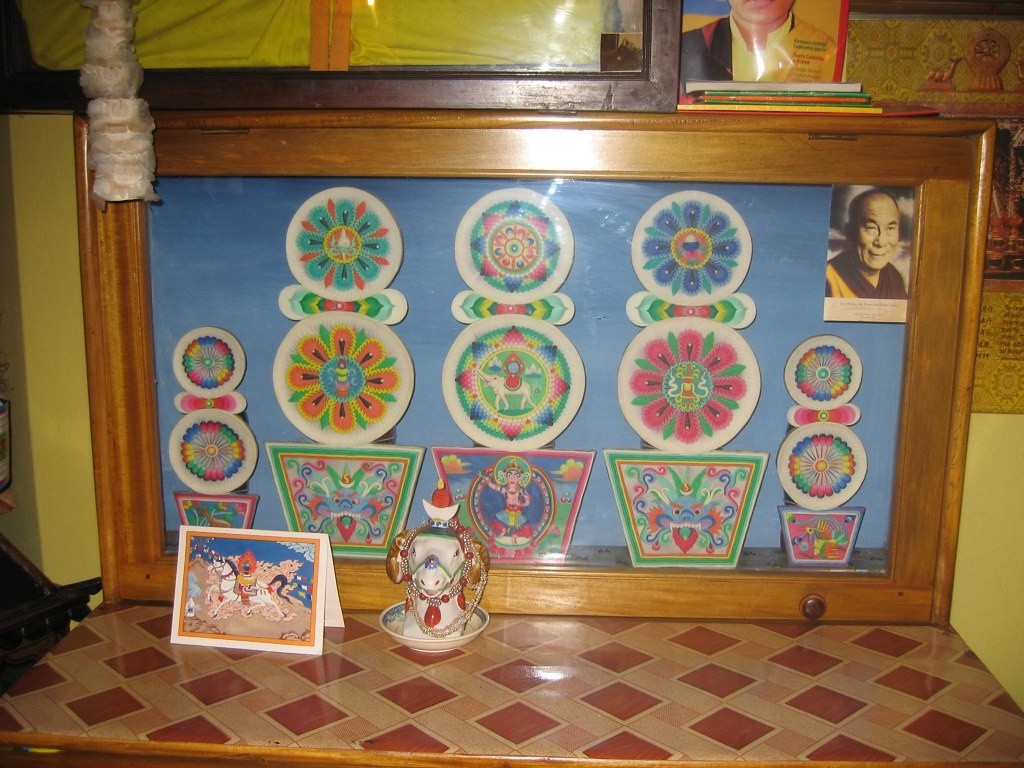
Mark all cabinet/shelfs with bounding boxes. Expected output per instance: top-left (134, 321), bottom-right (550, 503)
top-left (2, 0), bottom-right (679, 115)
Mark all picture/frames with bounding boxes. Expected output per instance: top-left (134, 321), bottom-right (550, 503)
top-left (72, 118), bottom-right (999, 626)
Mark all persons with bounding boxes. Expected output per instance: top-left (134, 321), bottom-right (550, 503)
top-left (824, 190), bottom-right (908, 300)
top-left (681, 2), bottom-right (837, 85)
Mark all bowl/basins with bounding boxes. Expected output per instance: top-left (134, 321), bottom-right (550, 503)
top-left (379, 598), bottom-right (490, 653)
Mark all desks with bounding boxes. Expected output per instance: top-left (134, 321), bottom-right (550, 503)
top-left (2, 605), bottom-right (1023, 768)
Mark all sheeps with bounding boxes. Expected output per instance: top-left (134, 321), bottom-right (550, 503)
top-left (385, 519), bottom-right (490, 641)
